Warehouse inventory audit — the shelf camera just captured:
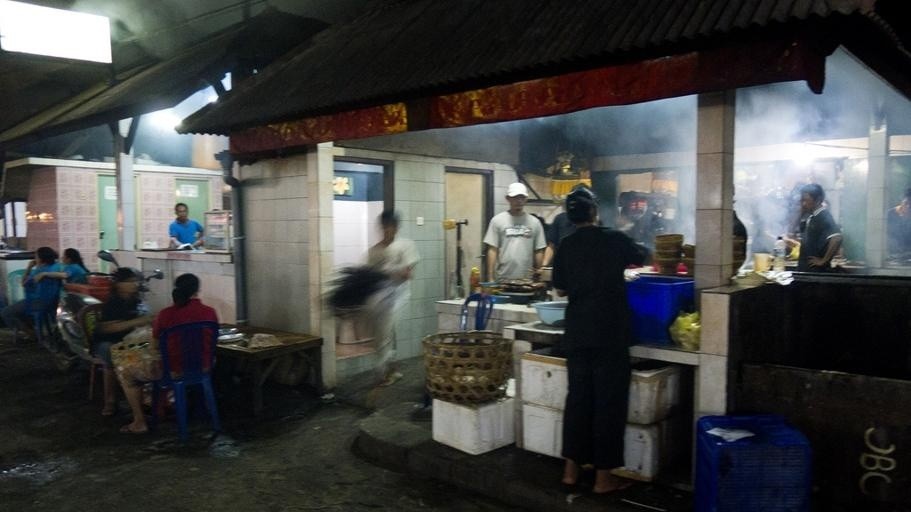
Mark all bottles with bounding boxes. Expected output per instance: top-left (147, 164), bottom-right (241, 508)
top-left (507, 183), bottom-right (528, 198)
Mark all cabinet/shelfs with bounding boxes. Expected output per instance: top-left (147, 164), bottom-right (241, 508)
top-left (774, 237), bottom-right (785, 272)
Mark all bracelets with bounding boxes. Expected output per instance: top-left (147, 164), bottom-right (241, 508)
top-left (680, 257), bottom-right (694, 276)
top-left (655, 234), bottom-right (683, 252)
top-left (530, 301), bottom-right (567, 327)
top-left (654, 252), bottom-right (681, 274)
top-left (682, 244), bottom-right (695, 258)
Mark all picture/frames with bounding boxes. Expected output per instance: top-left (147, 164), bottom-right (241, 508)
top-left (210, 323), bottom-right (323, 422)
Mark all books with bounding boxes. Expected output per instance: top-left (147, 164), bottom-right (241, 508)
top-left (431, 395), bottom-right (516, 457)
top-left (518, 345), bottom-right (681, 481)
top-left (625, 275), bottom-right (696, 350)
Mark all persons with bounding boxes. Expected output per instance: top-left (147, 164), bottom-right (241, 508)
top-left (542, 183), bottom-right (589, 267)
top-left (33, 248), bottom-right (91, 298)
top-left (88, 267), bottom-right (156, 418)
top-left (617, 190), bottom-right (679, 246)
top-left (483, 182), bottom-right (547, 285)
top-left (116, 273), bottom-right (219, 434)
top-left (682, 183), bottom-right (747, 272)
top-left (338, 210), bottom-right (421, 389)
top-left (551, 189), bottom-right (659, 501)
top-left (887, 188), bottom-right (911, 262)
top-left (0, 246), bottom-right (61, 344)
top-left (167, 203), bottom-right (205, 250)
top-left (784, 184), bottom-right (842, 273)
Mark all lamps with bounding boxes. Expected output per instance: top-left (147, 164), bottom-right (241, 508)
top-left (77, 303), bottom-right (118, 416)
top-left (6, 269), bottom-right (107, 343)
top-left (149, 320), bottom-right (221, 445)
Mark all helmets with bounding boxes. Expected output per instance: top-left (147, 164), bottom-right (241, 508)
top-left (218, 331), bottom-right (245, 343)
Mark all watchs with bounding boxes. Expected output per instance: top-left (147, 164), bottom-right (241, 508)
top-left (54, 249), bottom-right (164, 370)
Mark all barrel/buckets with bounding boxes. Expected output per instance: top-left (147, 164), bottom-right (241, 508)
top-left (119, 424), bottom-right (148, 435)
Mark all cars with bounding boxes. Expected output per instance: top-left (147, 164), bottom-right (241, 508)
top-left (752, 253), bottom-right (773, 272)
